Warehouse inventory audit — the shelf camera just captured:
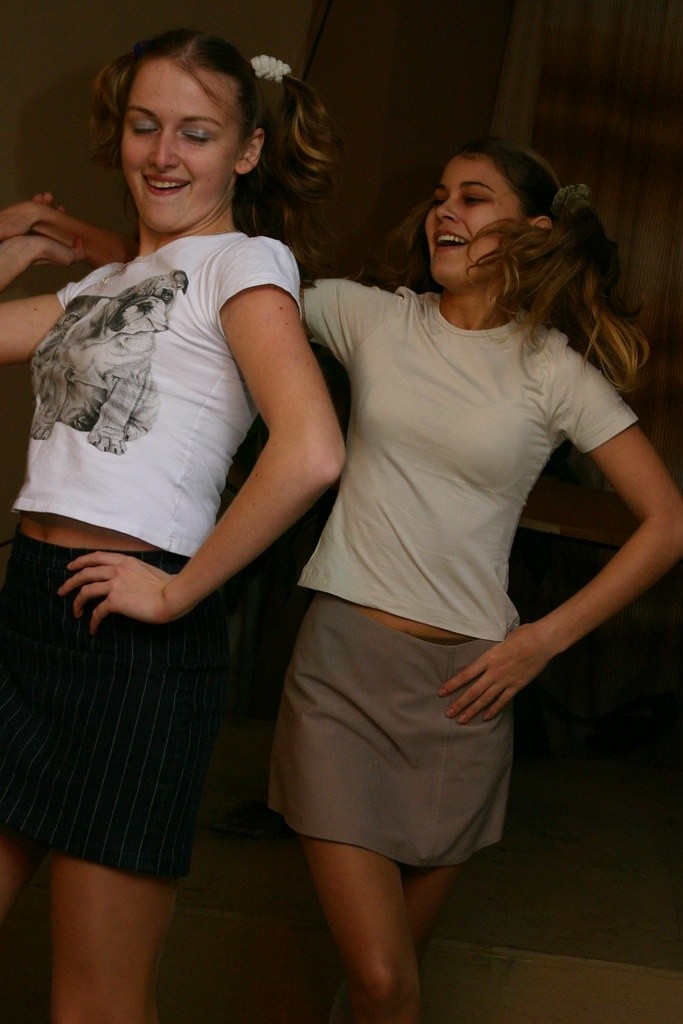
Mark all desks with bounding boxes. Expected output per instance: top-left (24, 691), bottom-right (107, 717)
top-left (225, 450), bottom-right (683, 735)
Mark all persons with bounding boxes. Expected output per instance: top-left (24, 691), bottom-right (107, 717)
top-left (1, 27), bottom-right (346, 1024)
top-left (32, 135), bottom-right (683, 1023)
